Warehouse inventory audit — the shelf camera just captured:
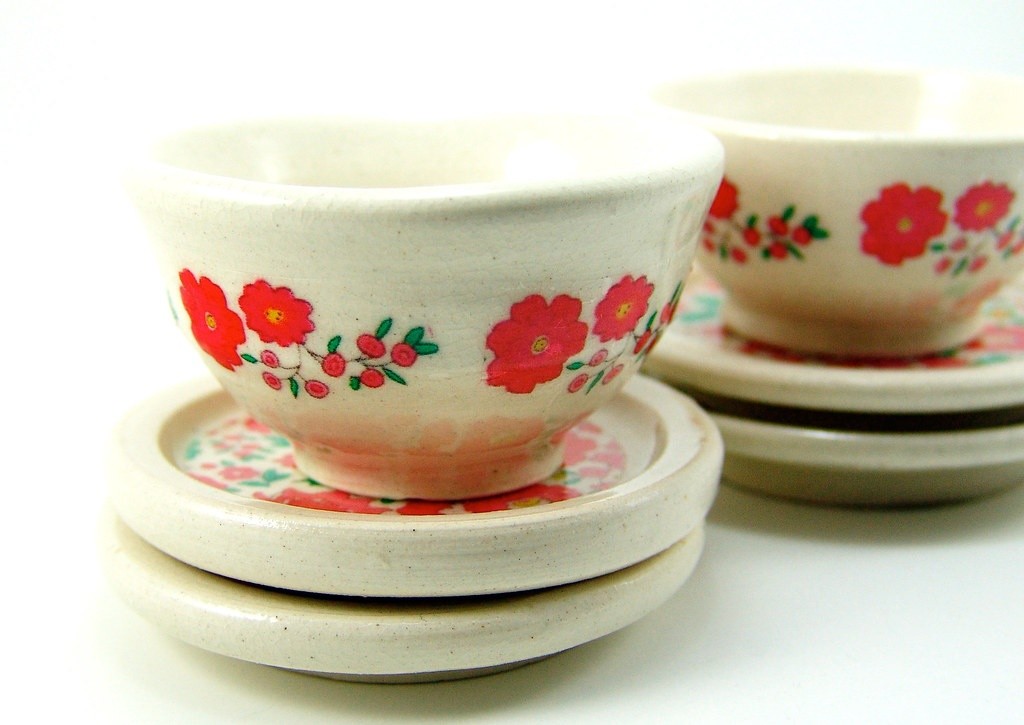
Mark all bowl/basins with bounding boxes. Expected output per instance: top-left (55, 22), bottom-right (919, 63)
top-left (690, 72), bottom-right (1024, 356)
top-left (139, 87), bottom-right (726, 511)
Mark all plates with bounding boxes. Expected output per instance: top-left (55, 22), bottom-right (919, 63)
top-left (116, 361), bottom-right (731, 683)
top-left (621, 284), bottom-right (1023, 533)
